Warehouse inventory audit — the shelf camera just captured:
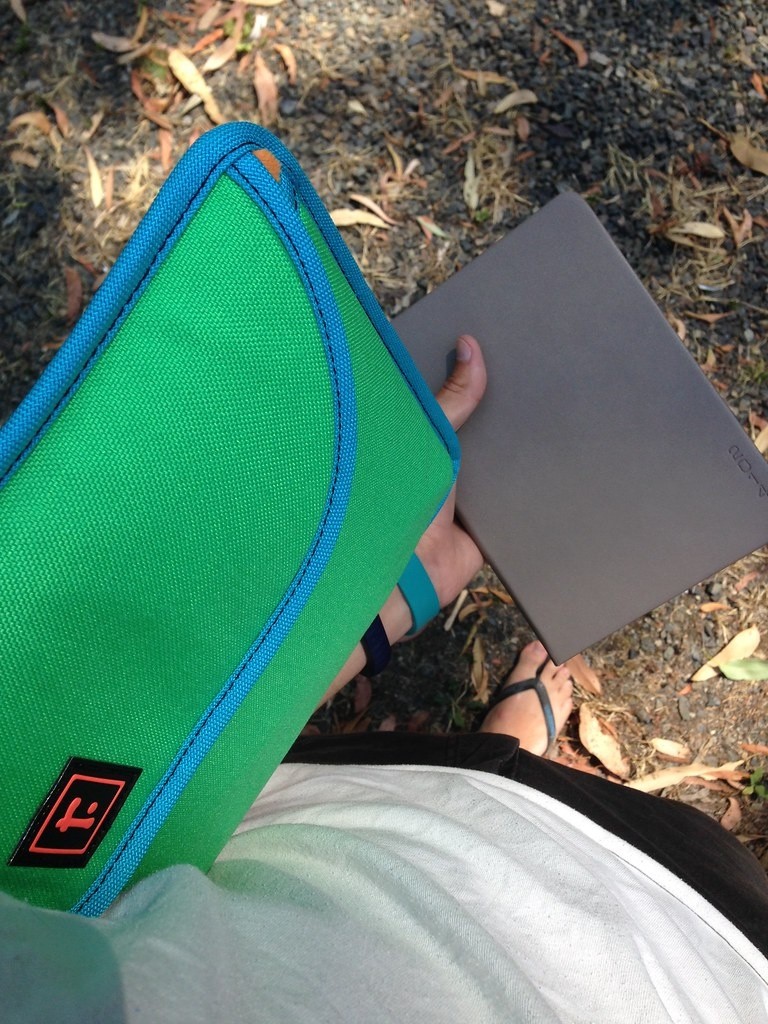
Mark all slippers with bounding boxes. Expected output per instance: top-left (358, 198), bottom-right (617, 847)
top-left (470, 646), bottom-right (556, 758)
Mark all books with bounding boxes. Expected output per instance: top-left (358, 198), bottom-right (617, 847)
top-left (385, 191), bottom-right (768, 669)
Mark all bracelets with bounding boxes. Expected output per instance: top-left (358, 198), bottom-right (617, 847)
top-left (358, 553), bottom-right (441, 664)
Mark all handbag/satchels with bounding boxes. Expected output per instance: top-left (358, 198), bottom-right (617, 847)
top-left (0, 121), bottom-right (462, 916)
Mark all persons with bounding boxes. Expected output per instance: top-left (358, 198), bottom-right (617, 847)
top-left (0, 335), bottom-right (768, 1024)
